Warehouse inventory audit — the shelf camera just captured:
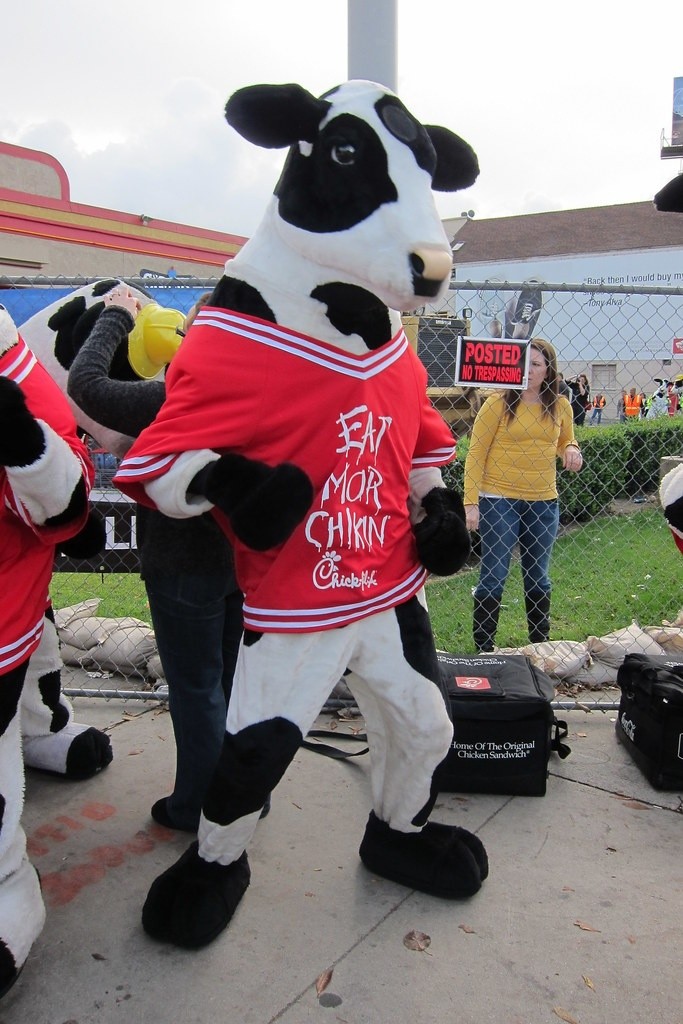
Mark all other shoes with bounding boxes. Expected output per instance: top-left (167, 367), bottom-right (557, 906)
top-left (152, 797), bottom-right (199, 833)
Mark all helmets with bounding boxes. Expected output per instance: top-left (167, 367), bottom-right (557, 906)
top-left (127, 303), bottom-right (187, 380)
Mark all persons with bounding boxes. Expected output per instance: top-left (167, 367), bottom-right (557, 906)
top-left (65, 287), bottom-right (248, 830)
top-left (556, 372), bottom-right (646, 426)
top-left (461, 336), bottom-right (582, 653)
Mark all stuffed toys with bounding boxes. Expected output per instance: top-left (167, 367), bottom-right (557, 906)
top-left (647, 375), bottom-right (683, 418)
top-left (113, 79), bottom-right (490, 954)
top-left (0, 277), bottom-right (166, 1003)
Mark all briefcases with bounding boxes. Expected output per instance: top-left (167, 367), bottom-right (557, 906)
top-left (615, 653), bottom-right (683, 790)
top-left (437, 654), bottom-right (571, 796)
